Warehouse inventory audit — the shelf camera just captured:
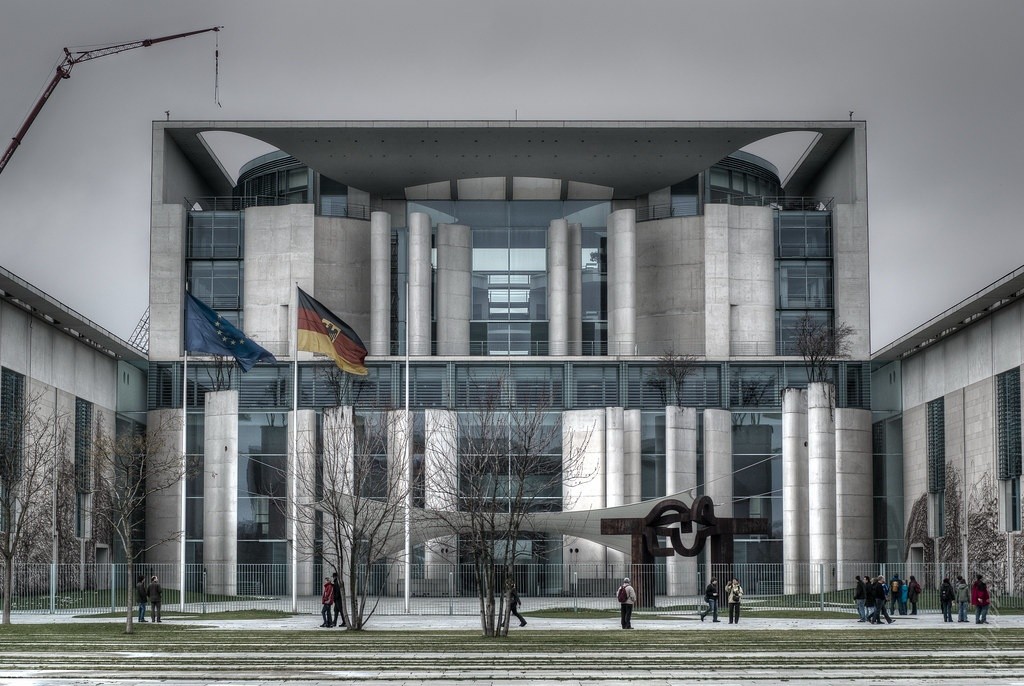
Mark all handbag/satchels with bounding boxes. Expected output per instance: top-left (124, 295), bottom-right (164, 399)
top-left (704, 593), bottom-right (709, 603)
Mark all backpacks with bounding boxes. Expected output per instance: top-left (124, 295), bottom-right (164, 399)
top-left (618, 585), bottom-right (629, 602)
top-left (914, 582), bottom-right (922, 594)
top-left (892, 581), bottom-right (899, 591)
top-left (941, 586), bottom-right (950, 601)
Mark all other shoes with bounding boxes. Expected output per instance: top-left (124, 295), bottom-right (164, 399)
top-left (138, 620), bottom-right (148, 622)
top-left (976, 621), bottom-right (983, 624)
top-left (889, 620), bottom-right (895, 624)
top-left (339, 624), bottom-right (346, 627)
top-left (157, 620), bottom-right (161, 622)
top-left (866, 616), bottom-right (884, 624)
top-left (330, 622), bottom-right (336, 627)
top-left (857, 619), bottom-right (865, 622)
top-left (152, 620), bottom-right (155, 622)
top-left (321, 623), bottom-right (333, 627)
top-left (713, 620), bottom-right (720, 622)
top-left (700, 613), bottom-right (704, 622)
top-left (983, 620), bottom-right (988, 624)
top-left (520, 622), bottom-right (527, 627)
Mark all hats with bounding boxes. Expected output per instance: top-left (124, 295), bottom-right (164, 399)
top-left (976, 574), bottom-right (983, 579)
top-left (331, 573), bottom-right (339, 577)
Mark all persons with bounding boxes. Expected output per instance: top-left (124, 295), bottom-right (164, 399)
top-left (502, 580), bottom-right (527, 627)
top-left (147, 576), bottom-right (162, 622)
top-left (700, 578), bottom-right (721, 622)
top-left (136, 576), bottom-right (149, 622)
top-left (890, 573), bottom-right (903, 615)
top-left (853, 576), bottom-right (866, 622)
top-left (907, 576), bottom-right (918, 615)
top-left (863, 575), bottom-right (896, 625)
top-left (955, 575), bottom-right (970, 622)
top-left (617, 578), bottom-right (637, 629)
top-left (971, 574), bottom-right (990, 624)
top-left (725, 578), bottom-right (743, 624)
top-left (940, 579), bottom-right (955, 622)
top-left (319, 577), bottom-right (334, 627)
top-left (901, 581), bottom-right (908, 615)
top-left (330, 573), bottom-right (347, 627)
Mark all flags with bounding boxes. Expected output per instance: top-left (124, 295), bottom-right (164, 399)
top-left (183, 292), bottom-right (278, 373)
top-left (295, 286), bottom-right (368, 375)
